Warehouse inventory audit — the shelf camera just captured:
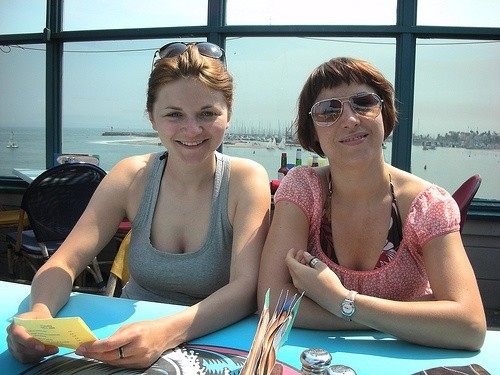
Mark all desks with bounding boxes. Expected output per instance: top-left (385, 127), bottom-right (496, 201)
top-left (0, 280), bottom-right (500, 375)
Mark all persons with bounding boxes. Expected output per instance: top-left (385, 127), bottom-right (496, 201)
top-left (6, 41), bottom-right (271, 370)
top-left (257, 56), bottom-right (487, 352)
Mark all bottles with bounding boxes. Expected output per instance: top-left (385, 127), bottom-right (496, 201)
top-left (296, 149), bottom-right (302, 166)
top-left (278, 153), bottom-right (289, 180)
top-left (300, 348), bottom-right (332, 375)
top-left (311, 154), bottom-right (319, 167)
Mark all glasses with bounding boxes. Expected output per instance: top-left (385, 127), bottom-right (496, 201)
top-left (152, 42), bottom-right (226, 72)
top-left (308, 93), bottom-right (385, 126)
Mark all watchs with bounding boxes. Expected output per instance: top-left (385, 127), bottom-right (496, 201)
top-left (339, 290), bottom-right (359, 322)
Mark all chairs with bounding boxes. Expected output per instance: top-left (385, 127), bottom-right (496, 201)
top-left (452, 174), bottom-right (482, 234)
top-left (5, 162), bottom-right (124, 298)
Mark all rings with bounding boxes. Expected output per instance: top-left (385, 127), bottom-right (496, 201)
top-left (308, 257), bottom-right (321, 268)
top-left (118, 346), bottom-right (124, 359)
